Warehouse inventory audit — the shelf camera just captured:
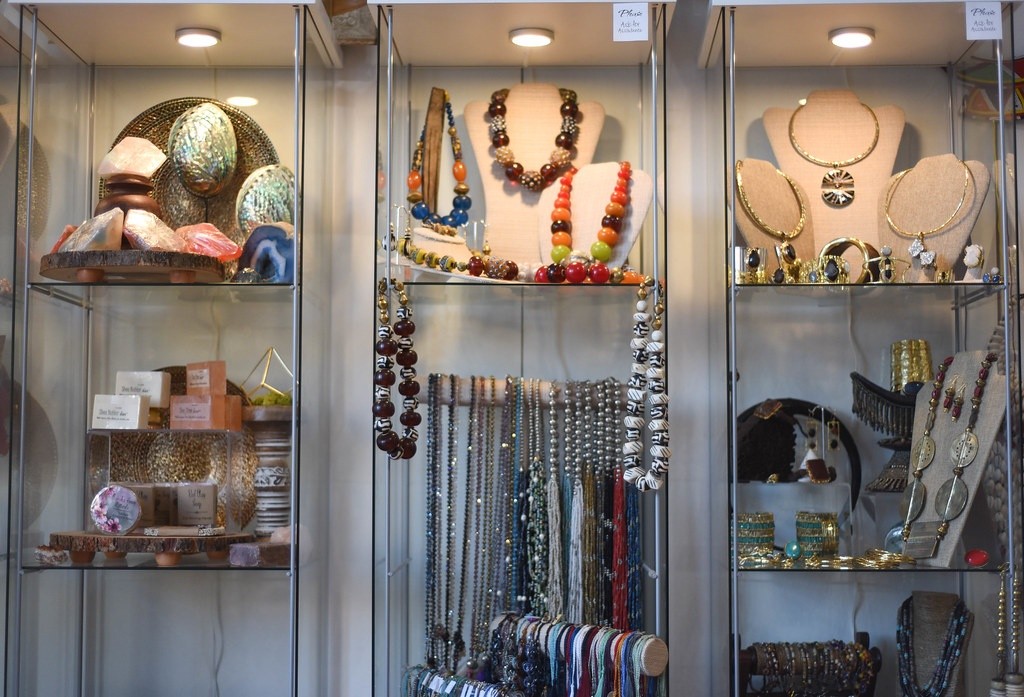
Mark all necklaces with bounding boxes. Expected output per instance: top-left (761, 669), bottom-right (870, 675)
top-left (370, 80), bottom-right (1023, 697)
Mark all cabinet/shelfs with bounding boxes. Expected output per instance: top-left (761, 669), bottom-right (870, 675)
top-left (0, 0), bottom-right (1023, 697)
